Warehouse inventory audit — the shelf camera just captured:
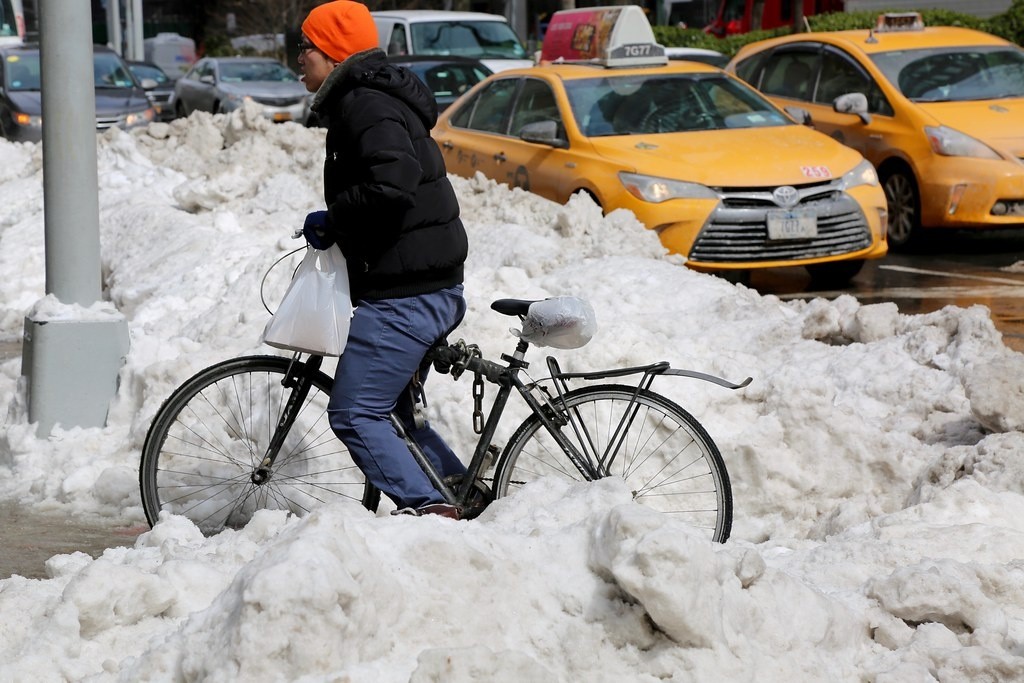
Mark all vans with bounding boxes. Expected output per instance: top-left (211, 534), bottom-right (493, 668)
top-left (369, 9), bottom-right (534, 118)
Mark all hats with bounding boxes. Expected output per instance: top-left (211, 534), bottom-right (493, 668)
top-left (301, 0), bottom-right (379, 63)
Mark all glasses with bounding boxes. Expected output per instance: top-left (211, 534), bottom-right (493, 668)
top-left (297, 43), bottom-right (316, 55)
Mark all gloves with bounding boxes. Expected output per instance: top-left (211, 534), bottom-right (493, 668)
top-left (303, 210), bottom-right (335, 251)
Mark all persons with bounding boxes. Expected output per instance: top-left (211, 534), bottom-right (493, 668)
top-left (293, 0), bottom-right (495, 525)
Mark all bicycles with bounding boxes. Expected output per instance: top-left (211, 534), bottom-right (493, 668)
top-left (140, 229), bottom-right (754, 550)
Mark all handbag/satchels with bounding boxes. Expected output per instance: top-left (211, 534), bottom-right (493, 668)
top-left (262, 242), bottom-right (351, 357)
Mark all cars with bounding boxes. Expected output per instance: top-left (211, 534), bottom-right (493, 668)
top-left (0, 41), bottom-right (160, 144)
top-left (712, 11), bottom-right (1024, 250)
top-left (429, 6), bottom-right (890, 288)
top-left (122, 58), bottom-right (177, 122)
top-left (174, 57), bottom-right (310, 125)
